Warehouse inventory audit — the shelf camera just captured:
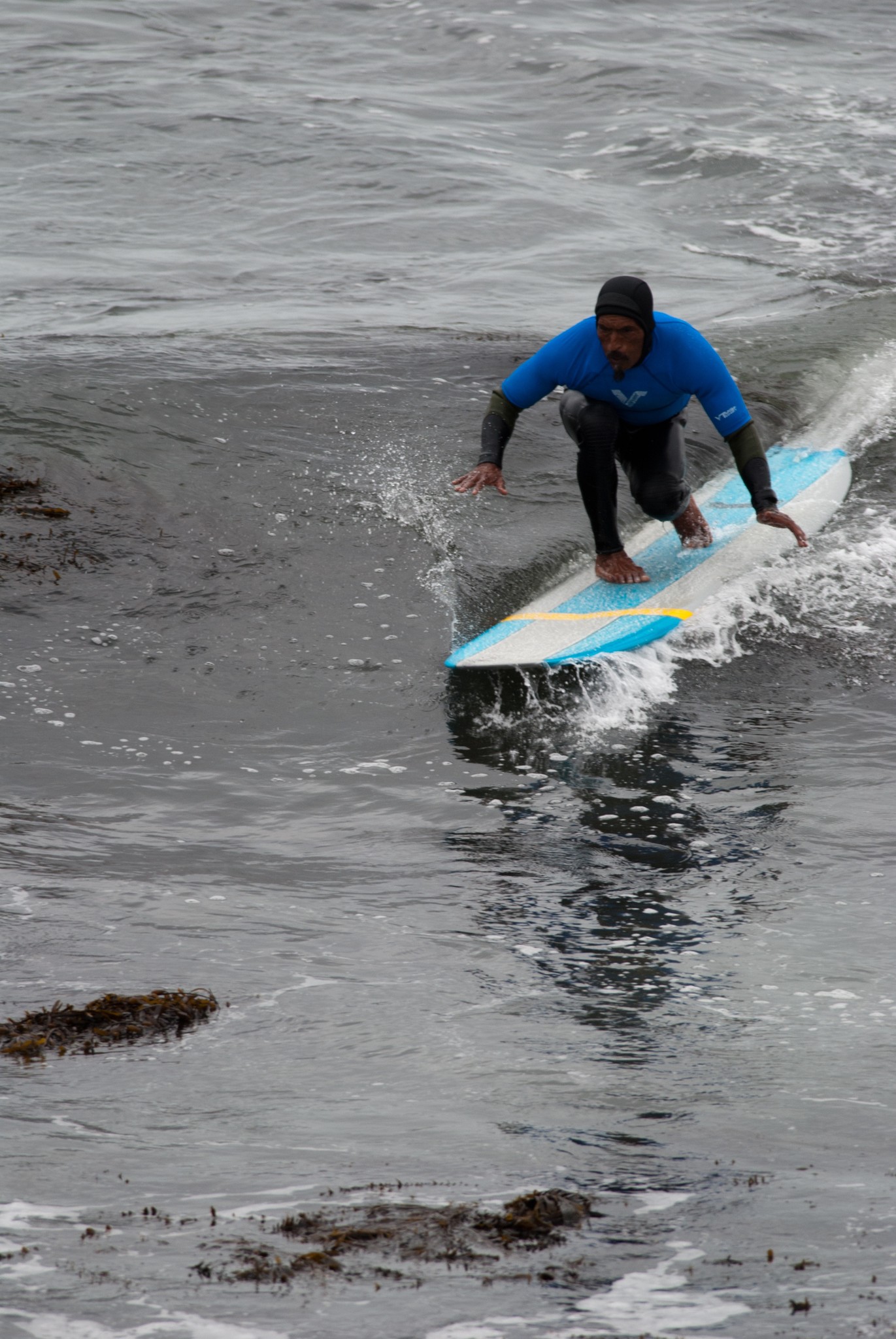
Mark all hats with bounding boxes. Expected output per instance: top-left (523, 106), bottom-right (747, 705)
top-left (595, 276), bottom-right (656, 339)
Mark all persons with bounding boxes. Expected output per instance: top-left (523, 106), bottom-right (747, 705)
top-left (452, 276), bottom-right (807, 584)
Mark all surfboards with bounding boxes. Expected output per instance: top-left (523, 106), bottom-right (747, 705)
top-left (442, 438), bottom-right (852, 667)
